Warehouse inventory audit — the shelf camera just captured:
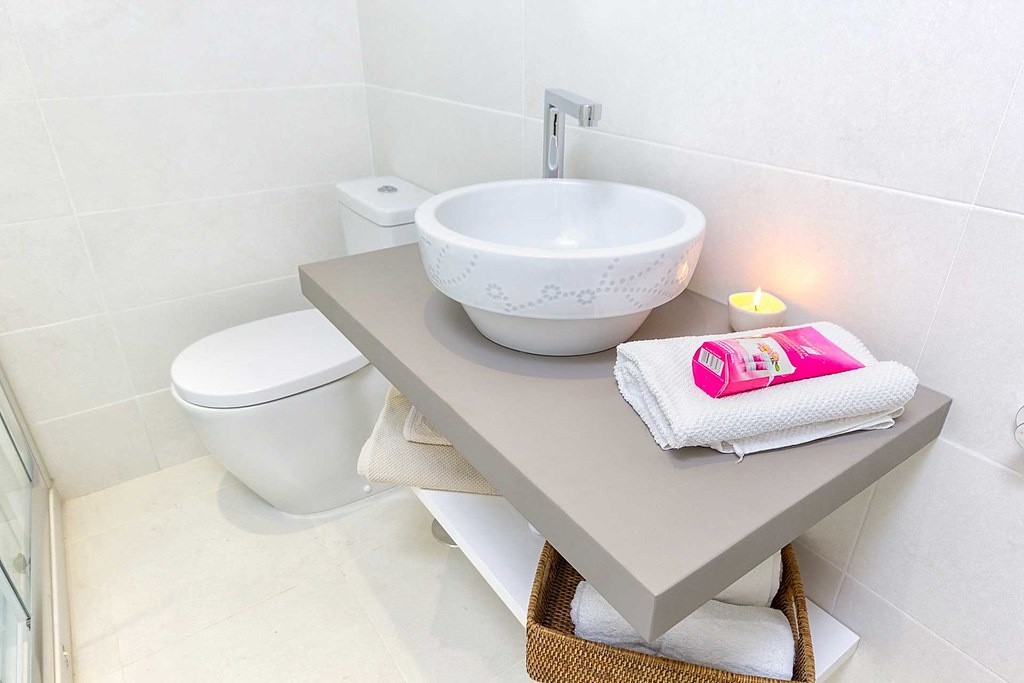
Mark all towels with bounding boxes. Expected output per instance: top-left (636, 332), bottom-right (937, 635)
top-left (355, 384), bottom-right (507, 498)
top-left (564, 548), bottom-right (800, 681)
top-left (613, 315), bottom-right (920, 465)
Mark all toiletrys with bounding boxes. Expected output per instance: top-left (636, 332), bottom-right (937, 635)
top-left (690, 326), bottom-right (867, 400)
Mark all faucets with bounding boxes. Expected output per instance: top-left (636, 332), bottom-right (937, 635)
top-left (539, 85), bottom-right (604, 181)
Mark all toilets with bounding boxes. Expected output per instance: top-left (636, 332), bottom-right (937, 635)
top-left (169, 173), bottom-right (439, 516)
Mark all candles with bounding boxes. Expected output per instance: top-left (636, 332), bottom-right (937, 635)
top-left (728, 286), bottom-right (787, 331)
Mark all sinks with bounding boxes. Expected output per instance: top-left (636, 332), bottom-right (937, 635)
top-left (414, 173), bottom-right (708, 359)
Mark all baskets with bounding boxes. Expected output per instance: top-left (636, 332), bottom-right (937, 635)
top-left (526, 539), bottom-right (813, 683)
